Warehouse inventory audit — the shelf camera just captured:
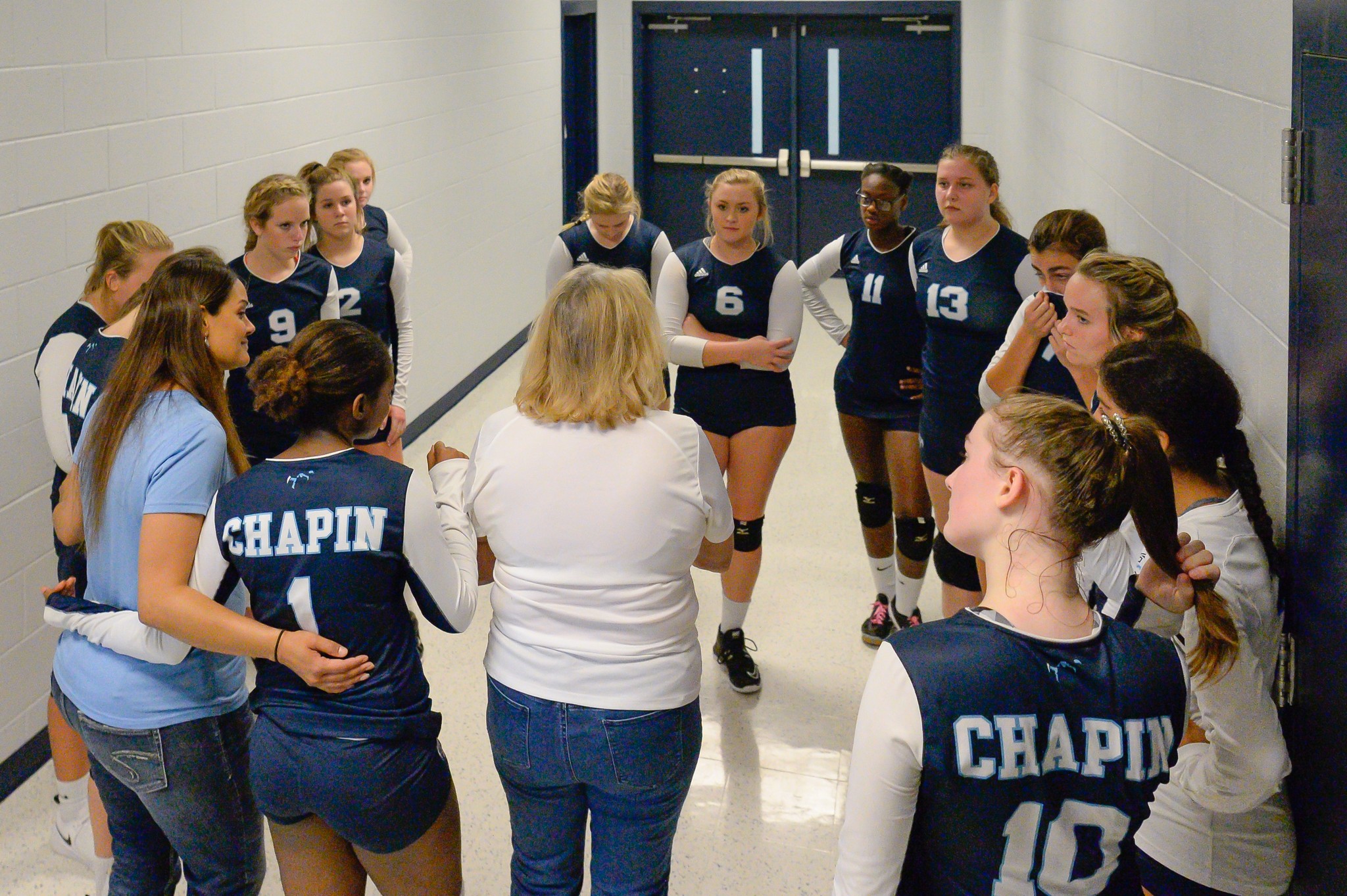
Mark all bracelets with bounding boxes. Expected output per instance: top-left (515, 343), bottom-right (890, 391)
top-left (275, 628), bottom-right (285, 664)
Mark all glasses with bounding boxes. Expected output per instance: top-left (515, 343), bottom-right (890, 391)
top-left (856, 187), bottom-right (906, 211)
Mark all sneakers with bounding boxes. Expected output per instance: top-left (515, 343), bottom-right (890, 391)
top-left (712, 623), bottom-right (762, 693)
top-left (860, 593), bottom-right (893, 646)
top-left (888, 595), bottom-right (922, 631)
top-left (52, 809), bottom-right (94, 866)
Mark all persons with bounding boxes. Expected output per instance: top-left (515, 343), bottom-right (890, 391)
top-left (34, 149), bottom-right (481, 896)
top-left (458, 262), bottom-right (732, 896)
top-left (549, 143), bottom-right (1295, 896)
